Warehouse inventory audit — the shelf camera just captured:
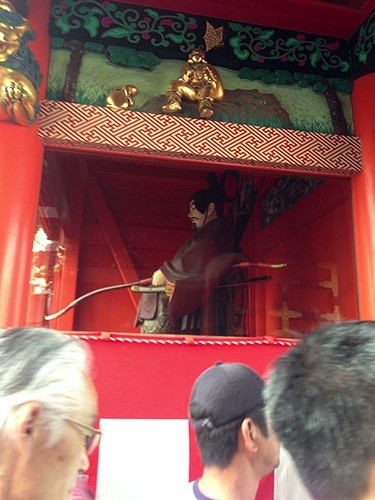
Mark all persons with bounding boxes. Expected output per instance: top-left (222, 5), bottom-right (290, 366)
top-left (0, 326), bottom-right (102, 500)
top-left (261, 320), bottom-right (375, 500)
top-left (134, 189), bottom-right (235, 333)
top-left (181, 362), bottom-right (281, 500)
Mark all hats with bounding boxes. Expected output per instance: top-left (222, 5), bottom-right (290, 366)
top-left (189, 361), bottom-right (267, 429)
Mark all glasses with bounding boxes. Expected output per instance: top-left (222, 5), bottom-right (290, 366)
top-left (64, 417), bottom-right (103, 454)
